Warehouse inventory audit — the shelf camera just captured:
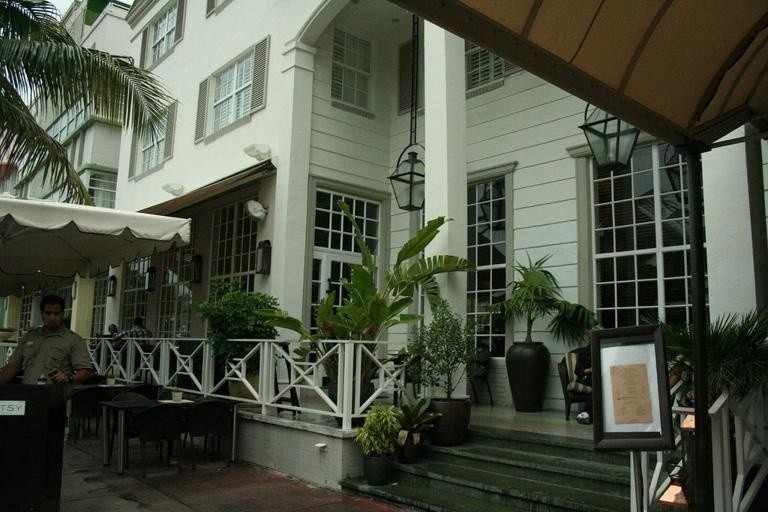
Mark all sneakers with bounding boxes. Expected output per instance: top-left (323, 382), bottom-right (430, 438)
top-left (576, 411), bottom-right (591, 424)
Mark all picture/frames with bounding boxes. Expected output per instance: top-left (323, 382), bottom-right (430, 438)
top-left (590, 322), bottom-right (675, 454)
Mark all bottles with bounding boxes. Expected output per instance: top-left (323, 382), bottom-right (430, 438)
top-left (37, 373), bottom-right (47, 384)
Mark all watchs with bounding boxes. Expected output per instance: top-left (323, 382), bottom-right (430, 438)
top-left (67, 375), bottom-right (73, 384)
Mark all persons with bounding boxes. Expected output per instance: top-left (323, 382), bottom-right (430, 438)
top-left (115, 317), bottom-right (152, 380)
top-left (0, 295), bottom-right (94, 385)
top-left (96, 324), bottom-right (122, 367)
top-left (575, 325), bottom-right (604, 425)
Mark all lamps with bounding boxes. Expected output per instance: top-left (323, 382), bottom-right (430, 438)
top-left (578, 102), bottom-right (641, 170)
top-left (190, 254), bottom-right (202, 282)
top-left (387, 14), bottom-right (426, 211)
top-left (144, 266), bottom-right (155, 291)
top-left (256, 240), bottom-right (271, 274)
top-left (106, 275), bottom-right (117, 295)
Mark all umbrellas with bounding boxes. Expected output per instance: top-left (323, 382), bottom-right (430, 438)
top-left (0, 193), bottom-right (193, 297)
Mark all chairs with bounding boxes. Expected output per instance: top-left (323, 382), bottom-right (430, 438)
top-left (65, 374), bottom-right (237, 476)
top-left (557, 348), bottom-right (600, 420)
top-left (388, 345), bottom-right (493, 409)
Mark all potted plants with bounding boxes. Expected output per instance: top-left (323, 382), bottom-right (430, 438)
top-left (196, 276), bottom-right (277, 403)
top-left (256, 201), bottom-right (476, 429)
top-left (392, 398), bottom-right (443, 463)
top-left (354, 405), bottom-right (404, 486)
top-left (483, 249), bottom-right (598, 414)
top-left (408, 301), bottom-right (482, 448)
